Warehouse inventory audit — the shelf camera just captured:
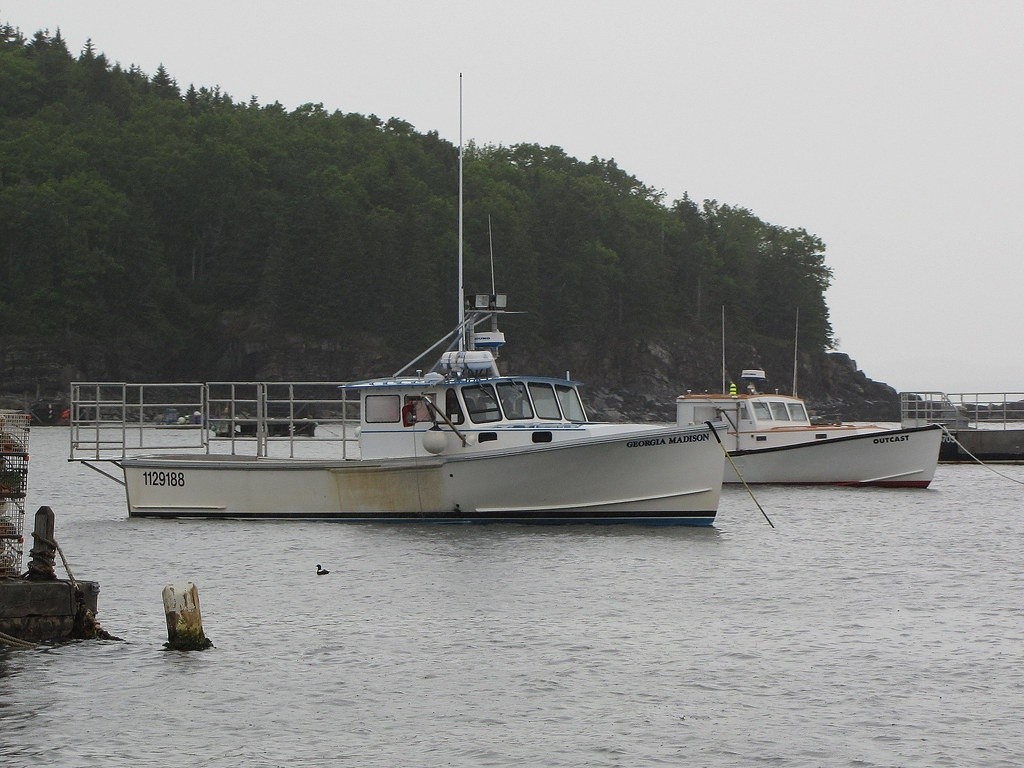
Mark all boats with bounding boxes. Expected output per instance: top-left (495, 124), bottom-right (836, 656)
top-left (212, 418), bottom-right (317, 438)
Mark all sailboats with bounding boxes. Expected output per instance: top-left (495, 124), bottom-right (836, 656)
top-left (675, 304), bottom-right (943, 489)
top-left (68, 73), bottom-right (731, 530)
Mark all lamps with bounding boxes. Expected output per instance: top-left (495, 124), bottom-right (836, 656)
top-left (467, 294), bottom-right (507, 308)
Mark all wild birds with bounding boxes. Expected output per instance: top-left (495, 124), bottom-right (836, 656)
top-left (315, 563), bottom-right (330, 575)
